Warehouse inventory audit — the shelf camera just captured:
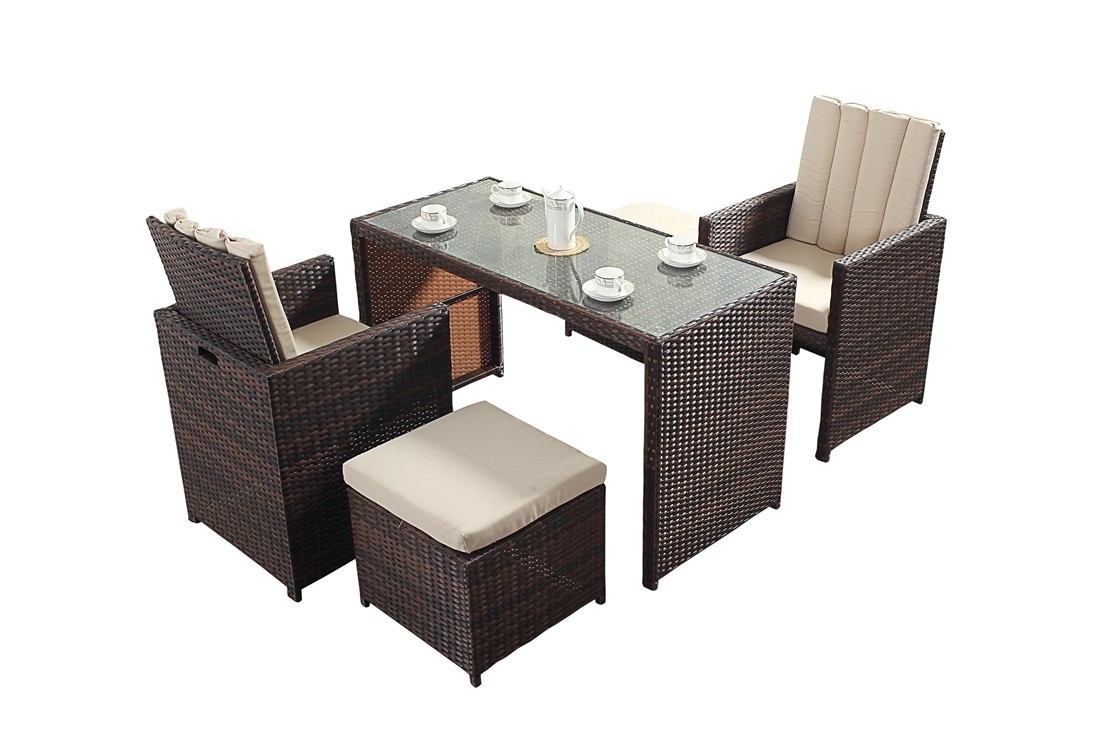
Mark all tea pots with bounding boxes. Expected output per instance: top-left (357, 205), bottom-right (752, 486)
top-left (542, 184), bottom-right (584, 251)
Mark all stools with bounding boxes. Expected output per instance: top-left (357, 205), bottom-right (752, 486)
top-left (340, 399), bottom-right (608, 690)
top-left (565, 200), bottom-right (706, 337)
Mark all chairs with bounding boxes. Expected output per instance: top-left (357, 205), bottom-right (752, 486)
top-left (700, 98), bottom-right (947, 463)
top-left (144, 210), bottom-right (454, 602)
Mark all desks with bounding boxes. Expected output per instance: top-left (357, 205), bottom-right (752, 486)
top-left (350, 177), bottom-right (798, 590)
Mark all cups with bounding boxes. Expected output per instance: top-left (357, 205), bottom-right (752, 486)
top-left (490, 180), bottom-right (524, 203)
top-left (664, 234), bottom-right (697, 263)
top-left (421, 204), bottom-right (448, 229)
top-left (594, 266), bottom-right (625, 297)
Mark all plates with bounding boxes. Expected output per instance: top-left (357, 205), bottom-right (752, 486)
top-left (581, 278), bottom-right (635, 302)
top-left (411, 215), bottom-right (458, 234)
top-left (488, 191), bottom-right (532, 208)
top-left (656, 247), bottom-right (708, 268)
